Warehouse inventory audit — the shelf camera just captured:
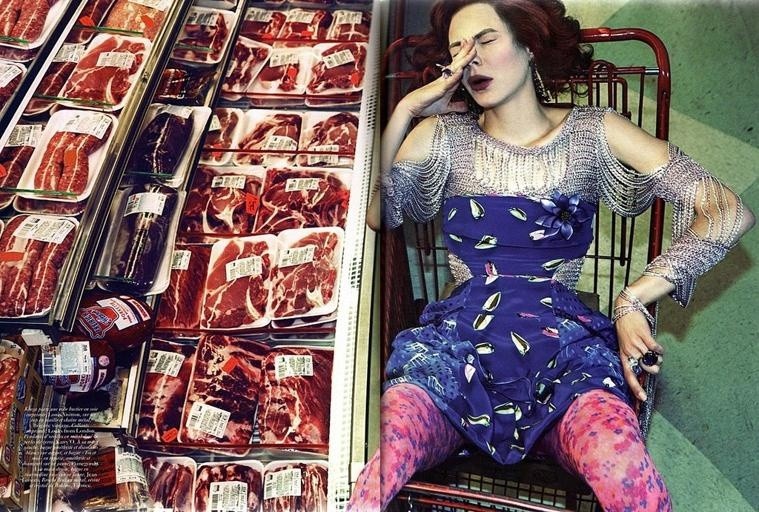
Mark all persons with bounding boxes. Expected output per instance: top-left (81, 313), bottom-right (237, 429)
top-left (346, 0), bottom-right (756, 511)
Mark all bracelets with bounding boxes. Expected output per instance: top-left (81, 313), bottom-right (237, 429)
top-left (610, 285), bottom-right (655, 327)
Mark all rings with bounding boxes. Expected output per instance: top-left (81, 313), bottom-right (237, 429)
top-left (435, 62), bottom-right (455, 79)
top-left (642, 350), bottom-right (658, 366)
top-left (626, 354), bottom-right (642, 375)
